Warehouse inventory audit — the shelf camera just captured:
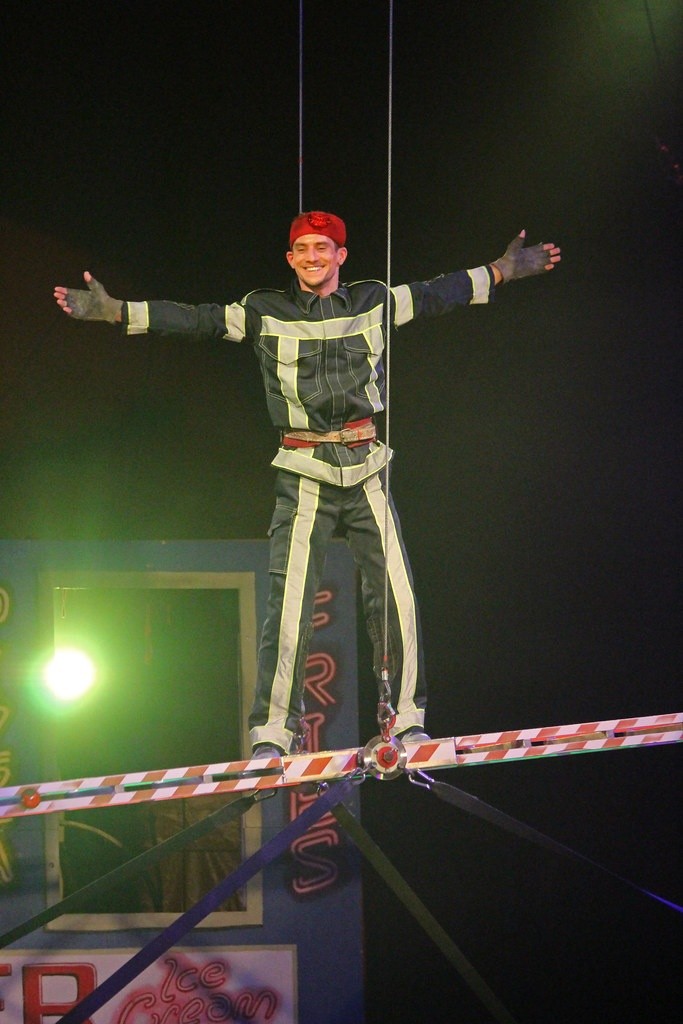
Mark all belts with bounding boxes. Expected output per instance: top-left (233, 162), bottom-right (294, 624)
top-left (283, 424), bottom-right (377, 443)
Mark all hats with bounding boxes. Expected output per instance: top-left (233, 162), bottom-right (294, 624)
top-left (289, 212), bottom-right (346, 248)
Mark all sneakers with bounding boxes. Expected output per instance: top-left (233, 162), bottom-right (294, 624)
top-left (400, 730), bottom-right (432, 742)
top-left (243, 746), bottom-right (283, 778)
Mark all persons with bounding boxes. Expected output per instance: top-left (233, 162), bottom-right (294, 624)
top-left (53, 211), bottom-right (561, 761)
